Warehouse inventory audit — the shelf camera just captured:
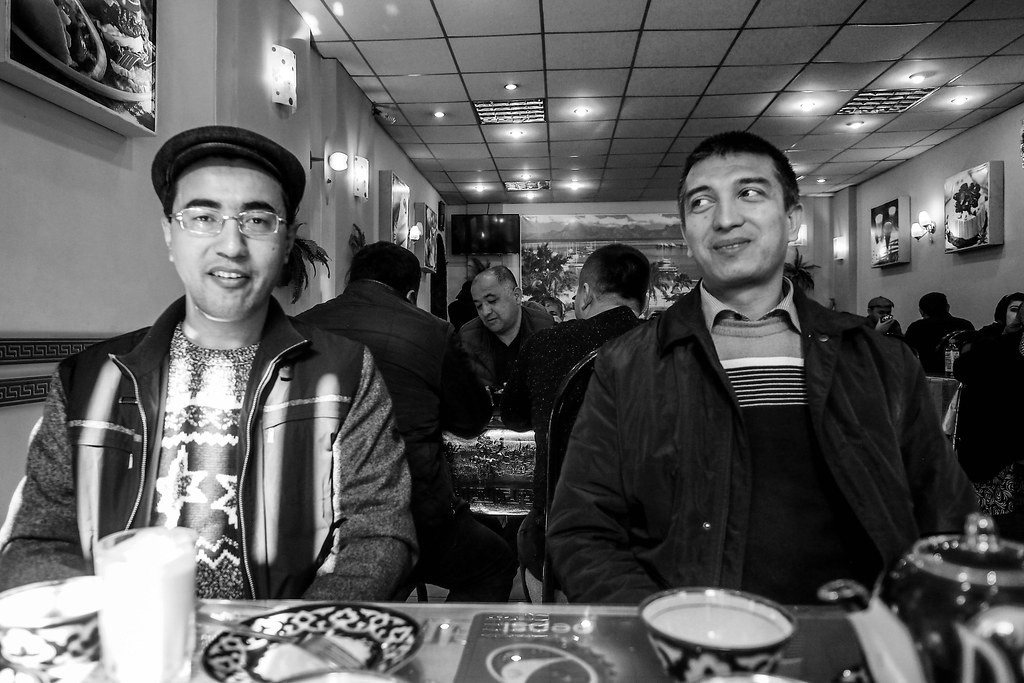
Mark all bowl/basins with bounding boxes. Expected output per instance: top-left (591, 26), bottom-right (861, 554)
top-left (881, 315), bottom-right (893, 321)
top-left (639, 586), bottom-right (799, 683)
top-left (0, 575), bottom-right (98, 672)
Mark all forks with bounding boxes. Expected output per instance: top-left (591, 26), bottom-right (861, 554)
top-left (194, 610), bottom-right (364, 670)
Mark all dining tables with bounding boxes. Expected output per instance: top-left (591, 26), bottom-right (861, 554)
top-left (193, 599), bottom-right (870, 683)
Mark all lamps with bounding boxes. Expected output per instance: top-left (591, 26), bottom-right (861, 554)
top-left (309, 139), bottom-right (349, 205)
top-left (911, 211), bottom-right (936, 244)
top-left (833, 236), bottom-right (845, 260)
top-left (409, 225), bottom-right (420, 246)
top-left (270, 44), bottom-right (298, 113)
top-left (354, 155), bottom-right (370, 200)
top-left (787, 224), bottom-right (807, 246)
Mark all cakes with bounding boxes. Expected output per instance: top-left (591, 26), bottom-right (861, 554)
top-left (947, 182), bottom-right (987, 240)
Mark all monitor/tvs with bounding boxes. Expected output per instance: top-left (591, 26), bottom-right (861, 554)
top-left (451, 214), bottom-right (520, 254)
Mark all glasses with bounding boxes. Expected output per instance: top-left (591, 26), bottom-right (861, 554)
top-left (169, 207), bottom-right (287, 239)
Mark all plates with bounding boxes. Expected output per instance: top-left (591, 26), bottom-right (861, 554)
top-left (202, 602), bottom-right (424, 683)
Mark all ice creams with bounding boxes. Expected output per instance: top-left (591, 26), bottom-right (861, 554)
top-left (883, 222), bottom-right (892, 249)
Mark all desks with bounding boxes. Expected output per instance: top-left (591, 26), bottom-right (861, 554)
top-left (923, 376), bottom-right (959, 453)
top-left (444, 429), bottom-right (536, 516)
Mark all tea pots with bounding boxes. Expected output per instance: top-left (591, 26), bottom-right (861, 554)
top-left (816, 513), bottom-right (1024, 683)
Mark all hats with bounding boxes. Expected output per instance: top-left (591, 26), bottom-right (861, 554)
top-left (868, 296), bottom-right (894, 307)
top-left (152, 126), bottom-right (306, 217)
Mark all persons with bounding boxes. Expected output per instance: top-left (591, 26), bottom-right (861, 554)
top-left (286, 241), bottom-right (518, 604)
top-left (0, 125), bottom-right (420, 601)
top-left (448, 279), bottom-right (478, 328)
top-left (902, 291), bottom-right (976, 377)
top-left (458, 265), bottom-right (556, 386)
top-left (498, 243), bottom-right (653, 595)
top-left (952, 292), bottom-right (1024, 544)
top-left (782, 262), bottom-right (814, 299)
top-left (543, 130), bottom-right (995, 605)
top-left (866, 296), bottom-right (903, 338)
top-left (535, 295), bottom-right (566, 324)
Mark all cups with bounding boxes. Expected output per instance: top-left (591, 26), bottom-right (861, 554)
top-left (92, 528), bottom-right (196, 683)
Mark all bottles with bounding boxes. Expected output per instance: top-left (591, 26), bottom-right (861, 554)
top-left (945, 338), bottom-right (960, 378)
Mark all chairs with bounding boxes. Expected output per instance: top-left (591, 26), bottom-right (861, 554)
top-left (397, 344), bottom-right (601, 604)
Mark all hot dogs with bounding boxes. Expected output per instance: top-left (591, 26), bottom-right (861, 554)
top-left (10, 0), bottom-right (108, 82)
